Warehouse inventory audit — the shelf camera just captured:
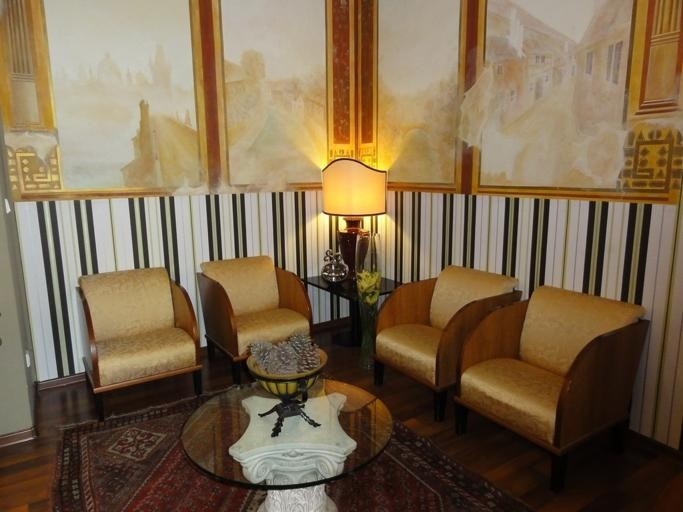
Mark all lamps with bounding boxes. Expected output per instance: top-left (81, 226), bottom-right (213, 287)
top-left (315, 156), bottom-right (388, 281)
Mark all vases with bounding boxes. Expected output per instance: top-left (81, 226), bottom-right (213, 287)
top-left (348, 231), bottom-right (385, 377)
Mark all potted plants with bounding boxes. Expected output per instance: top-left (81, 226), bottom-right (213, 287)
top-left (245, 327), bottom-right (328, 398)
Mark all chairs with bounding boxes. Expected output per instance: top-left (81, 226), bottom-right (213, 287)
top-left (75, 265), bottom-right (204, 424)
top-left (374, 263), bottom-right (524, 424)
top-left (196, 255), bottom-right (314, 383)
top-left (452, 282), bottom-right (651, 497)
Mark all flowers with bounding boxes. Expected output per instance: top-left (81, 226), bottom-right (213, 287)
top-left (354, 269), bottom-right (384, 368)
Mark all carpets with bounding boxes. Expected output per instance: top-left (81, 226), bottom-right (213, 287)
top-left (48, 368), bottom-right (539, 511)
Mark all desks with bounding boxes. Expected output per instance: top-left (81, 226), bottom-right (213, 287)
top-left (298, 274), bottom-right (405, 350)
top-left (176, 377), bottom-right (393, 512)
